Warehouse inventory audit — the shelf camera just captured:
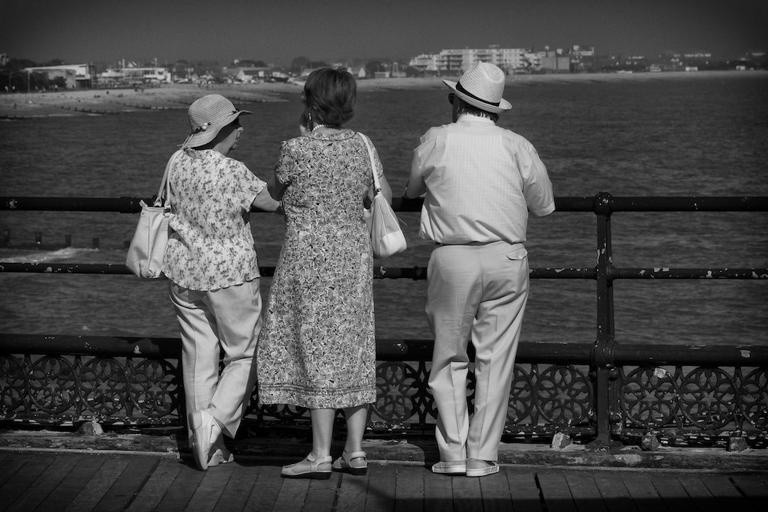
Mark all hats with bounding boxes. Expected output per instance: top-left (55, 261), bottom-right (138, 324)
top-left (444, 62), bottom-right (513, 114)
top-left (177, 94), bottom-right (252, 149)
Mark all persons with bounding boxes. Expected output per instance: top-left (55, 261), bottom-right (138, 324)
top-left (165, 94), bottom-right (282, 471)
top-left (255, 68), bottom-right (392, 477)
top-left (405, 60), bottom-right (556, 478)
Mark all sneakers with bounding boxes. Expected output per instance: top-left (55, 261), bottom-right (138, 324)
top-left (432, 458), bottom-right (499, 476)
top-left (189, 411), bottom-right (234, 470)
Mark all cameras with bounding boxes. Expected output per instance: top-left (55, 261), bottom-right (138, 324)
top-left (303, 112), bottom-right (312, 124)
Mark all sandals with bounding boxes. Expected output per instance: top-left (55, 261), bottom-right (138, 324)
top-left (281, 451), bottom-right (368, 479)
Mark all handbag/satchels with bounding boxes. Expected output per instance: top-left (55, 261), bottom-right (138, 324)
top-left (357, 192), bottom-right (407, 258)
top-left (126, 198), bottom-right (172, 279)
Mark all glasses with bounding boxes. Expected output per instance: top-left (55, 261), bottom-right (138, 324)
top-left (448, 93), bottom-right (455, 105)
